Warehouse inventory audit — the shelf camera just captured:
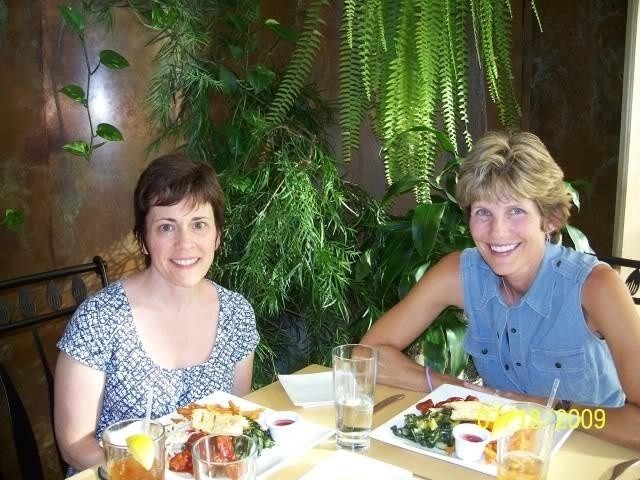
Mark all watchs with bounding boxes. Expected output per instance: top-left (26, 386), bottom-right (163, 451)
top-left (554, 399), bottom-right (572, 412)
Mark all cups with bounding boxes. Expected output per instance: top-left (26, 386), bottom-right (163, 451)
top-left (495, 402), bottom-right (556, 480)
top-left (332, 344), bottom-right (377, 452)
top-left (101, 418), bottom-right (257, 480)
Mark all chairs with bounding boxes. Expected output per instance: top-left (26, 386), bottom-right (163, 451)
top-left (0, 256), bottom-right (110, 479)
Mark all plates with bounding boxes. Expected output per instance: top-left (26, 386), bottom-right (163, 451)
top-left (371, 383), bottom-right (585, 477)
top-left (100, 389), bottom-right (334, 480)
top-left (276, 369), bottom-right (331, 409)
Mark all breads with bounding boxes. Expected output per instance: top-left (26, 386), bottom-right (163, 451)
top-left (443, 400), bottom-right (494, 421)
top-left (192, 409), bottom-right (244, 436)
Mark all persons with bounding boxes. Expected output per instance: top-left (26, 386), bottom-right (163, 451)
top-left (52, 153), bottom-right (262, 480)
top-left (349, 128), bottom-right (640, 453)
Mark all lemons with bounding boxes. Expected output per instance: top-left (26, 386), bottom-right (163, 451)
top-left (492, 410), bottom-right (524, 436)
top-left (126, 435), bottom-right (155, 470)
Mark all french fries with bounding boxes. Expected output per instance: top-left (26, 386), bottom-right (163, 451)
top-left (177, 400), bottom-right (266, 419)
top-left (485, 430), bottom-right (537, 463)
